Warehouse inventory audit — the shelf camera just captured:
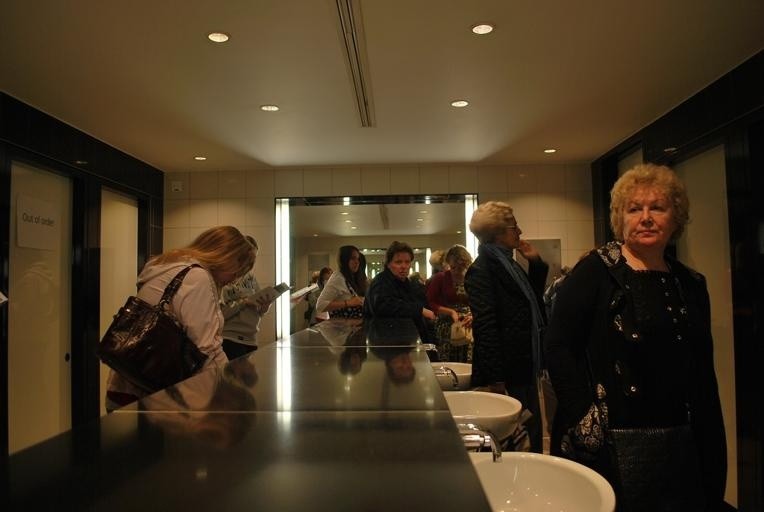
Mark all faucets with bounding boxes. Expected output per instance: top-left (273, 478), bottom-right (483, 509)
top-left (460, 429), bottom-right (503, 463)
top-left (417, 342), bottom-right (439, 353)
top-left (434, 366), bottom-right (460, 391)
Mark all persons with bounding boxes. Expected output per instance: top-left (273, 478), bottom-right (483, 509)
top-left (223, 353), bottom-right (258, 388)
top-left (463, 202), bottom-right (550, 454)
top-left (539, 164), bottom-right (728, 512)
top-left (289, 240), bottom-right (475, 364)
top-left (140, 366), bottom-right (256, 451)
top-left (215, 234), bottom-right (273, 362)
top-left (537, 249), bottom-right (592, 438)
top-left (312, 317), bottom-right (367, 375)
top-left (365, 317), bottom-right (420, 384)
top-left (542, 265), bottom-right (571, 314)
top-left (104, 226), bottom-right (257, 417)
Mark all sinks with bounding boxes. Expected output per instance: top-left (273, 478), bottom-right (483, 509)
top-left (466, 451), bottom-right (618, 512)
top-left (431, 362), bottom-right (473, 391)
top-left (445, 391), bottom-right (524, 442)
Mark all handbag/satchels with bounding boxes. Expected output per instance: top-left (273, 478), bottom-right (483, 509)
top-left (97, 296), bottom-right (209, 395)
top-left (330, 306), bottom-right (363, 320)
top-left (608, 426), bottom-right (703, 510)
top-left (450, 322), bottom-right (474, 344)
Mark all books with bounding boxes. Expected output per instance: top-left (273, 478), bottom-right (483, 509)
top-left (246, 281), bottom-right (290, 311)
